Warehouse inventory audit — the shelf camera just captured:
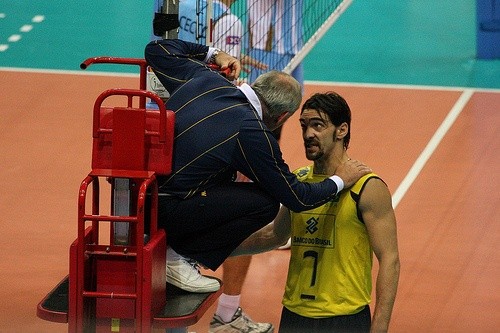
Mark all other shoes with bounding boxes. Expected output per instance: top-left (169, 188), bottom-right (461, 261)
top-left (165, 248), bottom-right (218, 293)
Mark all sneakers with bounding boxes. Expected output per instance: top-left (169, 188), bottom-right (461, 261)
top-left (210, 306), bottom-right (272, 333)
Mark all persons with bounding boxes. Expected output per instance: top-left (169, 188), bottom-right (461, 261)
top-left (226, 91), bottom-right (401, 333)
top-left (145, 0), bottom-right (372, 332)
